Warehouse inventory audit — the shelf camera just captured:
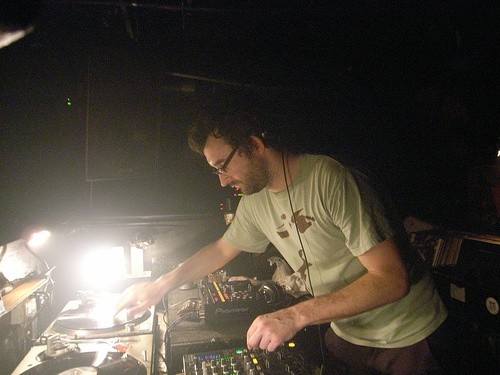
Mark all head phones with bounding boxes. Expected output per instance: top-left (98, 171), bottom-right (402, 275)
top-left (247, 281), bottom-right (282, 304)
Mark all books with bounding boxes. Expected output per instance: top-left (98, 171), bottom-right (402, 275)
top-left (409, 229), bottom-right (460, 266)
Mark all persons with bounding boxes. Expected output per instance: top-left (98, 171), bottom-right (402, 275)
top-left (108, 94), bottom-right (451, 375)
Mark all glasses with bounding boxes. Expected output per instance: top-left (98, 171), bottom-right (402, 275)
top-left (213, 146), bottom-right (238, 176)
